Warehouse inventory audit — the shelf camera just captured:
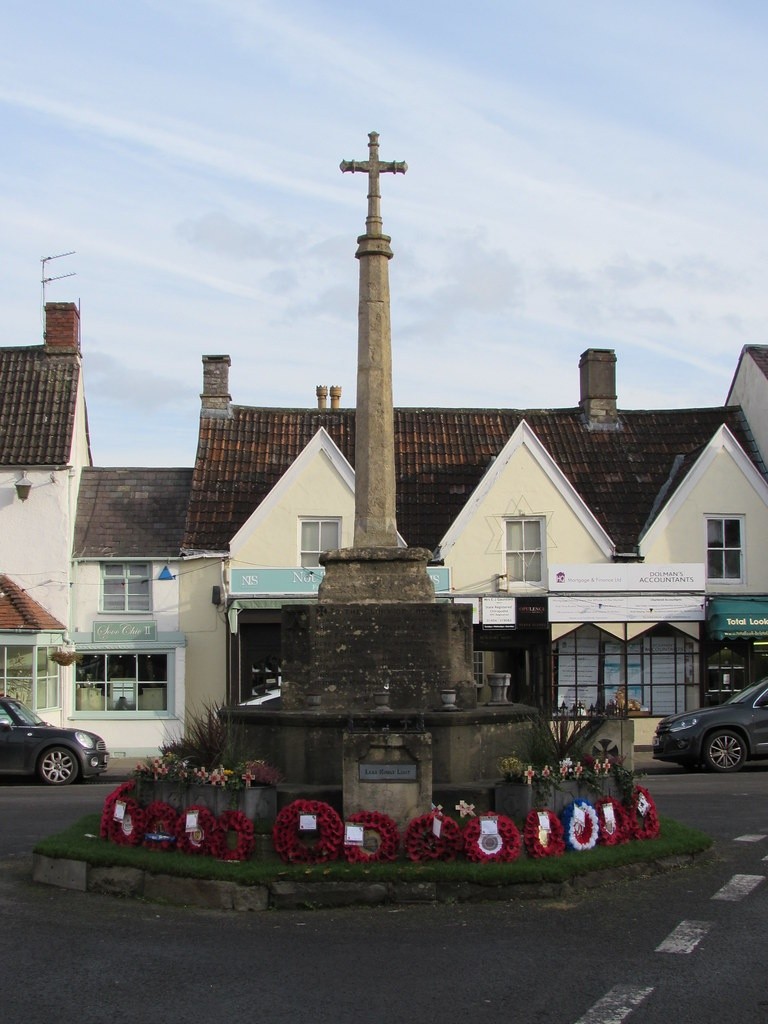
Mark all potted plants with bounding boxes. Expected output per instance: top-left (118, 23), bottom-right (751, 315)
top-left (134, 695), bottom-right (288, 836)
top-left (49, 652), bottom-right (84, 667)
top-left (493, 706), bottom-right (636, 831)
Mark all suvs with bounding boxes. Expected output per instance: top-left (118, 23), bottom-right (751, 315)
top-left (0, 696), bottom-right (110, 785)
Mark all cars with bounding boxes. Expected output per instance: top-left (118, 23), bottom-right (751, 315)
top-left (653, 674), bottom-right (767, 772)
top-left (217, 688), bottom-right (284, 718)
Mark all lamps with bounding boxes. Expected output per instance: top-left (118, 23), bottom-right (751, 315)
top-left (15, 470), bottom-right (33, 502)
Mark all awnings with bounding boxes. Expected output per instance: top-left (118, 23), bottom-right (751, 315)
top-left (707, 597), bottom-right (768, 641)
